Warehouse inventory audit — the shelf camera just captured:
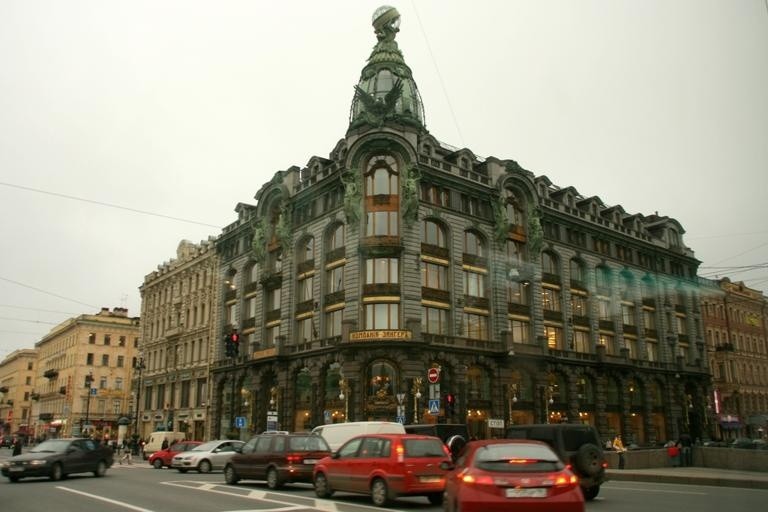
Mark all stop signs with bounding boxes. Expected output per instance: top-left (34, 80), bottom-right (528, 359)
top-left (427, 368), bottom-right (438, 383)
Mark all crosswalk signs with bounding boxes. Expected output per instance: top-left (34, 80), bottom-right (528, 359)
top-left (429, 400), bottom-right (440, 415)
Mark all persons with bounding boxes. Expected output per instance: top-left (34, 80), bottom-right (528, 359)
top-left (496, 196), bottom-right (510, 237)
top-left (83, 428), bottom-right (134, 465)
top-left (251, 228), bottom-right (265, 257)
top-left (674, 427), bottom-right (694, 467)
top-left (401, 171), bottom-right (424, 221)
top-left (605, 432), bottom-right (627, 469)
top-left (338, 169), bottom-right (359, 220)
top-left (469, 432), bottom-right (480, 441)
top-left (11, 433), bottom-right (22, 456)
top-left (276, 213), bottom-right (291, 246)
top-left (532, 210), bottom-right (543, 250)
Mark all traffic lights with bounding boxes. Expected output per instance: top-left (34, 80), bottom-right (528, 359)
top-left (140, 440), bottom-right (145, 445)
top-left (84, 376), bottom-right (90, 387)
top-left (224, 329), bottom-right (240, 357)
top-left (445, 393), bottom-right (454, 418)
top-left (123, 438), bottom-right (130, 445)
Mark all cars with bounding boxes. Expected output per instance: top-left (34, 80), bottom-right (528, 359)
top-left (0, 438), bottom-right (115, 481)
top-left (697, 434), bottom-right (768, 450)
top-left (0, 435), bottom-right (11, 450)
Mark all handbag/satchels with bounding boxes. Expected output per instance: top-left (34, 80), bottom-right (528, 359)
top-left (668, 447), bottom-right (679, 457)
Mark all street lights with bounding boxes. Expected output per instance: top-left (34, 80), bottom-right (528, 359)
top-left (270, 388), bottom-right (279, 412)
top-left (684, 394), bottom-right (693, 424)
top-left (338, 378), bottom-right (349, 422)
top-left (411, 378), bottom-right (422, 423)
top-left (241, 389), bottom-right (254, 428)
top-left (132, 349), bottom-right (145, 456)
top-left (508, 384), bottom-right (518, 424)
top-left (544, 385), bottom-right (554, 424)
